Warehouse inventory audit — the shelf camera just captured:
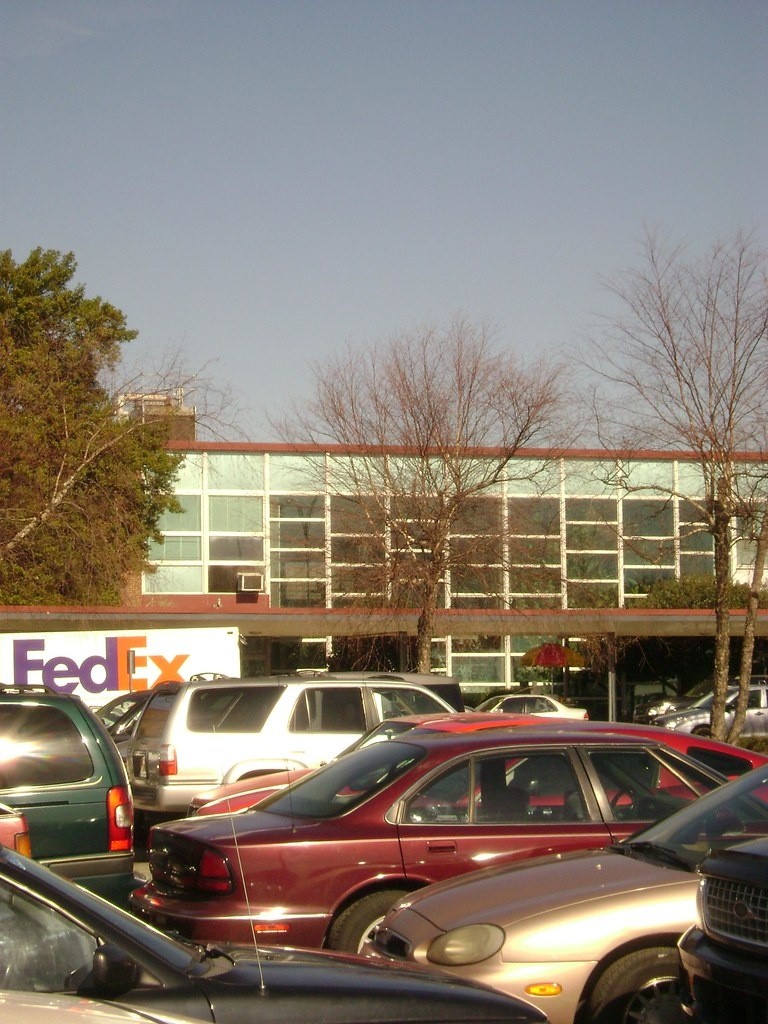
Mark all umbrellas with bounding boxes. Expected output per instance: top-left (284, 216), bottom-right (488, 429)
top-left (521, 642), bottom-right (584, 693)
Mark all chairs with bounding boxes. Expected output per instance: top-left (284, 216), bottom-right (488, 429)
top-left (494, 787), bottom-right (528, 819)
top-left (562, 791), bottom-right (585, 822)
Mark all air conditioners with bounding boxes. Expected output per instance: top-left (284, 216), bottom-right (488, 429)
top-left (237, 573), bottom-right (264, 591)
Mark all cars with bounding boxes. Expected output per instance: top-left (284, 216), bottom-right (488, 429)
top-left (354, 760), bottom-right (768, 1024)
top-left (0, 674), bottom-right (768, 901)
top-left (122, 728), bottom-right (768, 954)
top-left (672, 827), bottom-right (768, 1024)
top-left (0, 838), bottom-right (550, 1024)
